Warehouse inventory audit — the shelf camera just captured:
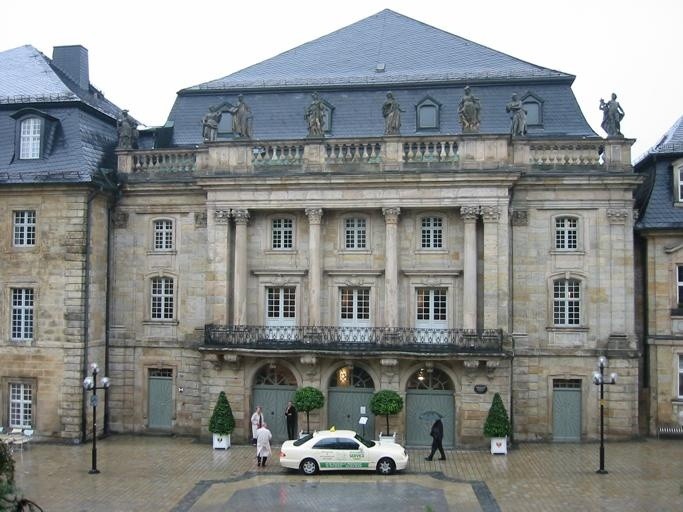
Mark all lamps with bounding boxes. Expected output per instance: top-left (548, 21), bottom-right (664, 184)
top-left (416, 371), bottom-right (424, 381)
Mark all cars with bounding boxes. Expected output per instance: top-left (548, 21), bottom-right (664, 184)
top-left (278, 426), bottom-right (410, 477)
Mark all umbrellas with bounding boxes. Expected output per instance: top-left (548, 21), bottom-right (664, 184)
top-left (418, 411), bottom-right (442, 421)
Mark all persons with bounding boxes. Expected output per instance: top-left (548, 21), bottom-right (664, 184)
top-left (256, 421), bottom-right (273, 467)
top-left (251, 406), bottom-right (264, 446)
top-left (305, 91), bottom-right (326, 135)
top-left (458, 85), bottom-right (478, 129)
top-left (232, 92), bottom-right (251, 136)
top-left (382, 91), bottom-right (406, 135)
top-left (284, 400), bottom-right (296, 440)
top-left (505, 92), bottom-right (526, 137)
top-left (423, 418), bottom-right (445, 460)
top-left (116, 109), bottom-right (144, 150)
top-left (201, 105), bottom-right (222, 142)
top-left (600, 92), bottom-right (625, 136)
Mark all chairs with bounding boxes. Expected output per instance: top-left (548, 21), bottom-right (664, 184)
top-left (0, 428), bottom-right (37, 453)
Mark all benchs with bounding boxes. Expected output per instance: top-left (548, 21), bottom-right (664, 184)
top-left (657, 421), bottom-right (683, 440)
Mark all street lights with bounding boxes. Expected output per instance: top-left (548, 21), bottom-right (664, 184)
top-left (591, 356), bottom-right (618, 473)
top-left (82, 360), bottom-right (112, 475)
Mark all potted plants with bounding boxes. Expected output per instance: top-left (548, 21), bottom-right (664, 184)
top-left (210, 391), bottom-right (235, 451)
top-left (485, 391), bottom-right (510, 454)
top-left (292, 386), bottom-right (324, 439)
top-left (370, 391), bottom-right (402, 444)
